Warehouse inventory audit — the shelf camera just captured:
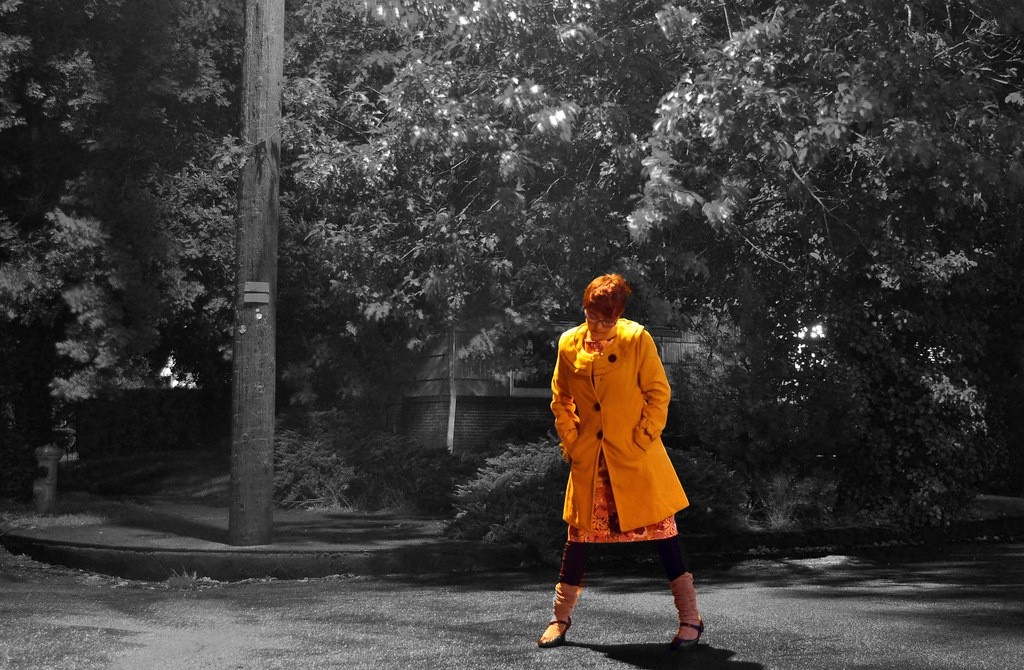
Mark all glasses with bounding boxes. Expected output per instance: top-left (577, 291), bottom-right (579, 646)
top-left (587, 309), bottom-right (619, 328)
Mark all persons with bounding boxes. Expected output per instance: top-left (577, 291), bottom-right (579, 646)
top-left (538, 274), bottom-right (704, 652)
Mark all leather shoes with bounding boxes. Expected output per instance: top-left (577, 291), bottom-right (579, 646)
top-left (539, 616), bottom-right (571, 647)
top-left (670, 620), bottom-right (704, 651)
